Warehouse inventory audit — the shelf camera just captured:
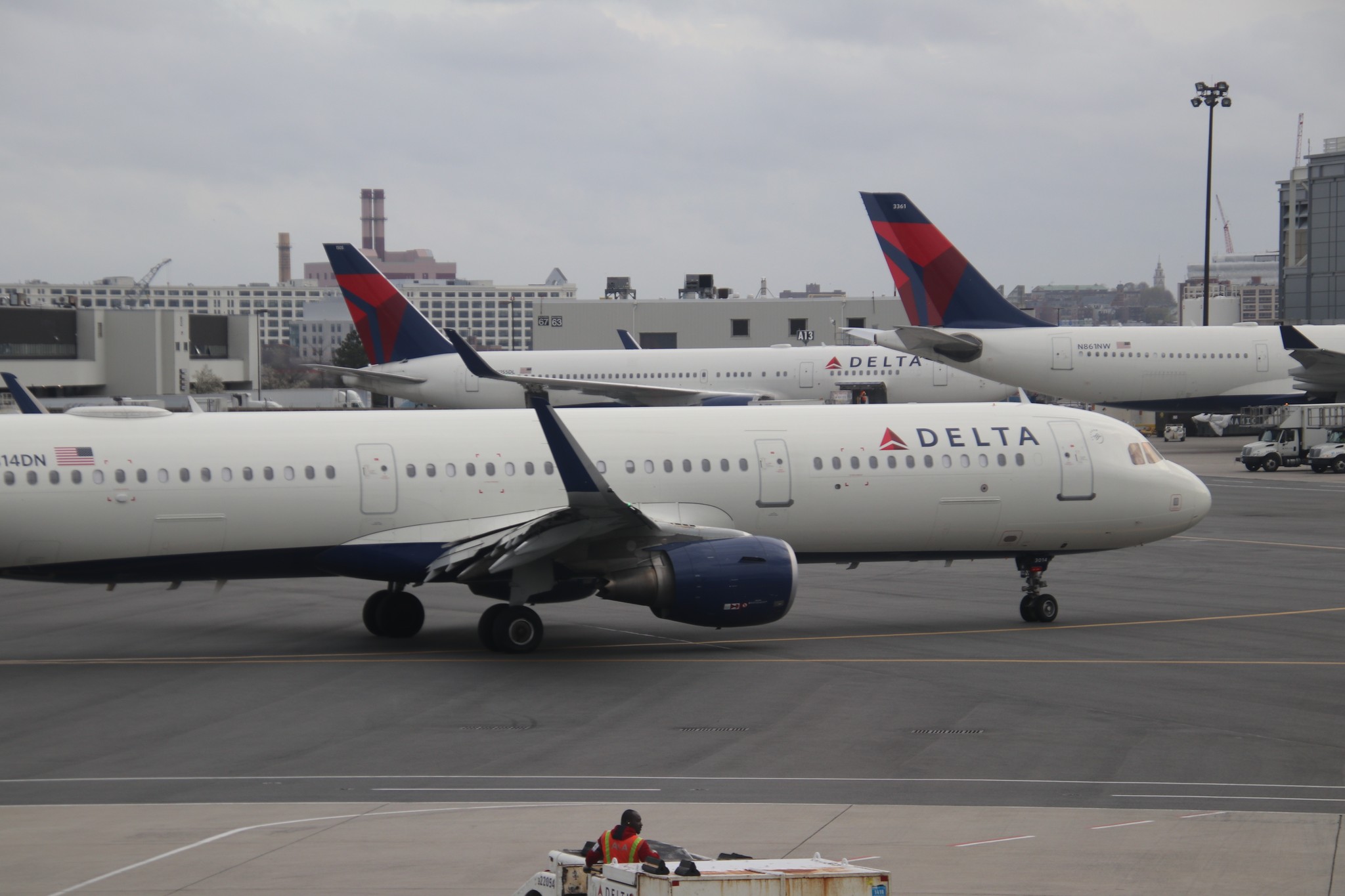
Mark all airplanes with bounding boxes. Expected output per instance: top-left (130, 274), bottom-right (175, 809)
top-left (300, 240), bottom-right (1055, 410)
top-left (0, 399), bottom-right (1213, 656)
top-left (839, 190), bottom-right (1345, 430)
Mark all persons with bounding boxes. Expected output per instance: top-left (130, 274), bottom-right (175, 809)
top-left (585, 809), bottom-right (660, 866)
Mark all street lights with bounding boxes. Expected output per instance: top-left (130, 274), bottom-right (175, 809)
top-left (1189, 78), bottom-right (1232, 328)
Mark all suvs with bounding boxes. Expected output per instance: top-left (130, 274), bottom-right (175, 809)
top-left (1306, 428), bottom-right (1345, 474)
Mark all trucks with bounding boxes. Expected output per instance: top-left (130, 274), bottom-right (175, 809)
top-left (1234, 402), bottom-right (1345, 472)
